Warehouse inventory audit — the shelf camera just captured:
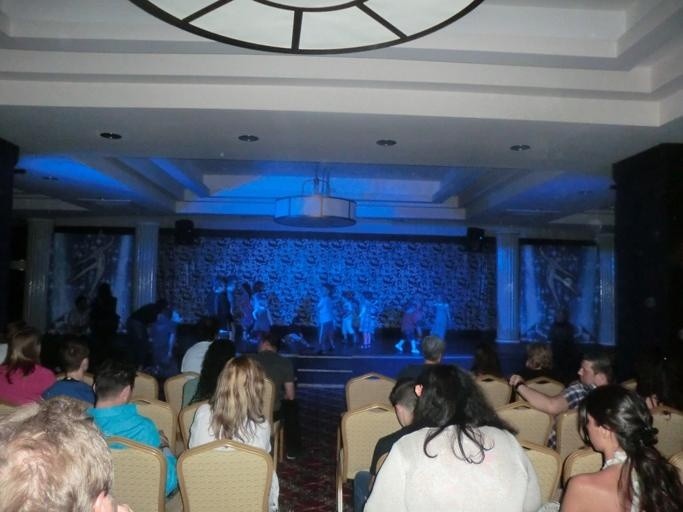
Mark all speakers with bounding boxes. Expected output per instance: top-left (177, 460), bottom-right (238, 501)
top-left (175, 220), bottom-right (193, 245)
top-left (177, 324), bottom-right (213, 357)
top-left (467, 227), bottom-right (484, 252)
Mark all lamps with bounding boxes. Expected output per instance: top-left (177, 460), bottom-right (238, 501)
top-left (271, 159), bottom-right (359, 229)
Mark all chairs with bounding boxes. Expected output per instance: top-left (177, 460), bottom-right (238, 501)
top-left (0, 339), bottom-right (289, 512)
top-left (332, 372), bottom-right (683, 511)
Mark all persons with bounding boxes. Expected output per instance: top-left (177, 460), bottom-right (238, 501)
top-left (208, 276), bottom-right (454, 354)
top-left (354, 336), bottom-right (682, 511)
top-left (1, 280), bottom-right (300, 512)
